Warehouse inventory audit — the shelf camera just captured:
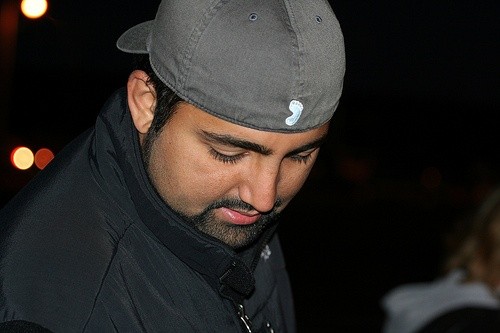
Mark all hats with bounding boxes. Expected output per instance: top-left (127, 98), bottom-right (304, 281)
top-left (116, 0), bottom-right (346, 133)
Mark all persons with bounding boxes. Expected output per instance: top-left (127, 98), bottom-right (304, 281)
top-left (0, 0), bottom-right (347, 332)
top-left (381, 185), bottom-right (500, 333)
